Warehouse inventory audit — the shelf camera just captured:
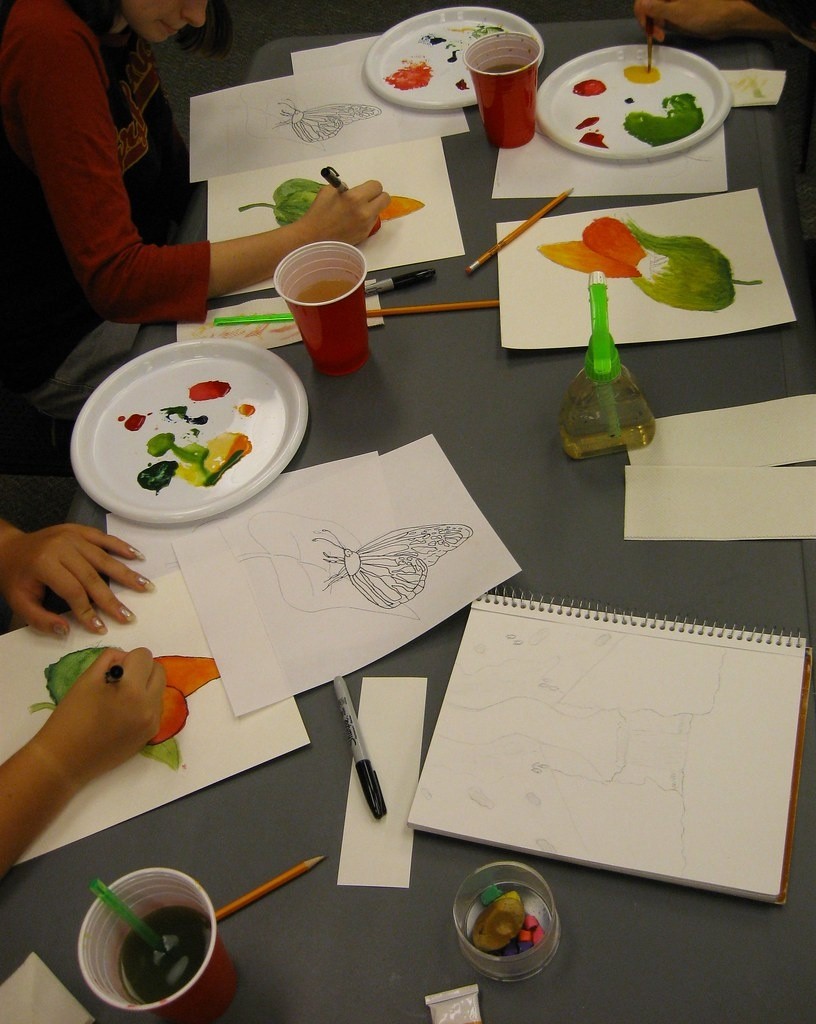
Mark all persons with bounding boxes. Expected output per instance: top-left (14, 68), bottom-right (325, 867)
top-left (0, 0), bottom-right (390, 458)
top-left (634, 0), bottom-right (816, 52)
top-left (1, 518), bottom-right (165, 879)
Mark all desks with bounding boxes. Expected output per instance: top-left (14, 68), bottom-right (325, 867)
top-left (0, 14), bottom-right (814, 1024)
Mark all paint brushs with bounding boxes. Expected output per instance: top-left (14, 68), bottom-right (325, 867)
top-left (645, 14), bottom-right (654, 74)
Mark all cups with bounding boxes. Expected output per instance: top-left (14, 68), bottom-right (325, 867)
top-left (462, 32), bottom-right (542, 149)
top-left (273, 241), bottom-right (371, 376)
top-left (78, 867), bottom-right (237, 1024)
top-left (453, 860), bottom-right (562, 980)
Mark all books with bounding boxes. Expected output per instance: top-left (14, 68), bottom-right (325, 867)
top-left (407, 585), bottom-right (813, 904)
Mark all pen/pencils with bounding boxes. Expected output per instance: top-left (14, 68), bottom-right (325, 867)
top-left (334, 675), bottom-right (386, 819)
top-left (363, 268), bottom-right (438, 297)
top-left (466, 187), bottom-right (575, 277)
top-left (214, 314), bottom-right (294, 326)
top-left (364, 301), bottom-right (500, 317)
top-left (105, 664), bottom-right (125, 683)
top-left (216, 854), bottom-right (327, 922)
top-left (321, 166), bottom-right (348, 193)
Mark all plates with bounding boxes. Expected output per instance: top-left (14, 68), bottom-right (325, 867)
top-left (365, 6), bottom-right (544, 108)
top-left (69, 338), bottom-right (308, 523)
top-left (535, 44), bottom-right (732, 159)
